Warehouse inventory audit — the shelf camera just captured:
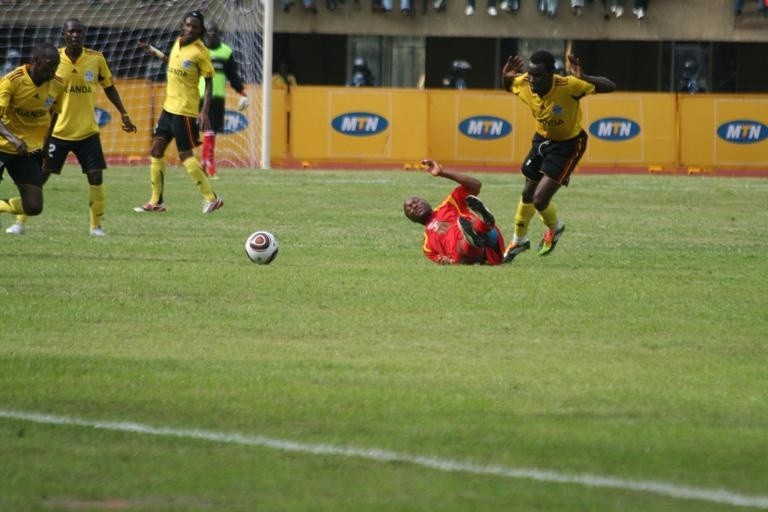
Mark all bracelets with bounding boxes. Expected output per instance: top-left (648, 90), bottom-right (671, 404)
top-left (121, 112), bottom-right (130, 117)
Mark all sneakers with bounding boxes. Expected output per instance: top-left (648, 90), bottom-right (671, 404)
top-left (203, 197), bottom-right (223, 213)
top-left (89, 228), bottom-right (107, 237)
top-left (205, 169), bottom-right (221, 179)
top-left (466, 195), bottom-right (495, 225)
top-left (501, 238), bottom-right (530, 263)
top-left (133, 202), bottom-right (165, 213)
top-left (537, 221), bottom-right (565, 255)
top-left (5, 222), bottom-right (25, 233)
top-left (458, 216), bottom-right (485, 248)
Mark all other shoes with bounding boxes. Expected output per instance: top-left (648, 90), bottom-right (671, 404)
top-left (304, 6), bottom-right (318, 14)
top-left (371, 3), bottom-right (644, 19)
top-left (327, 3), bottom-right (336, 10)
top-left (284, 4), bottom-right (290, 12)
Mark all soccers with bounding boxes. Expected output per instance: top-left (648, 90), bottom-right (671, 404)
top-left (245, 231), bottom-right (278, 264)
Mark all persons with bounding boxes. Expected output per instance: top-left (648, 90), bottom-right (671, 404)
top-left (0, 44), bottom-right (69, 216)
top-left (402, 158), bottom-right (506, 266)
top-left (4, 16), bottom-right (138, 238)
top-left (502, 48), bottom-right (617, 263)
top-left (195, 18), bottom-right (251, 181)
top-left (346, 56), bottom-right (380, 87)
top-left (554, 58), bottom-right (572, 76)
top-left (443, 56), bottom-right (471, 88)
top-left (273, 57), bottom-right (298, 112)
top-left (674, 56), bottom-right (709, 93)
top-left (131, 10), bottom-right (225, 214)
